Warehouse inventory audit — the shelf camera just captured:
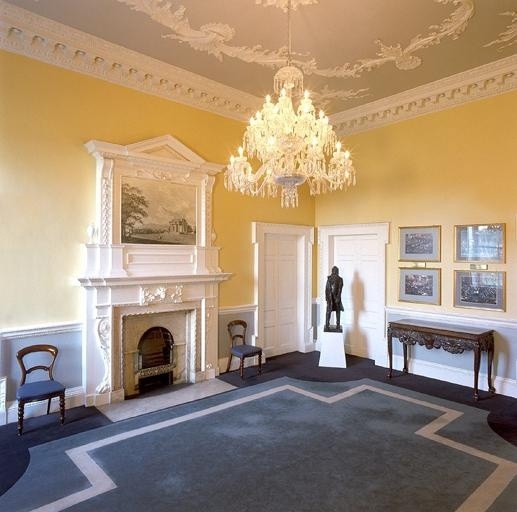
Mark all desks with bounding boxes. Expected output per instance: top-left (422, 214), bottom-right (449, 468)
top-left (386, 319), bottom-right (495, 403)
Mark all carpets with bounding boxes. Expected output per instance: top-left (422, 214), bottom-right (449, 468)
top-left (96, 377), bottom-right (239, 423)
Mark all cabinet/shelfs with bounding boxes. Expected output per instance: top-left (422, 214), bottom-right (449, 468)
top-left (397, 267), bottom-right (441, 306)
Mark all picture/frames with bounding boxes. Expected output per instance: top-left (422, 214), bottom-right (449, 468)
top-left (397, 225), bottom-right (441, 262)
top-left (453, 223), bottom-right (506, 264)
top-left (453, 270), bottom-right (506, 313)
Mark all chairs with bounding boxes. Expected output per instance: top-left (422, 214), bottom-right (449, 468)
top-left (16, 344), bottom-right (66, 437)
top-left (226, 320), bottom-right (262, 379)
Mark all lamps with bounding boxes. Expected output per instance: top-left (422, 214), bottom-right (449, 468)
top-left (223, 0), bottom-right (357, 208)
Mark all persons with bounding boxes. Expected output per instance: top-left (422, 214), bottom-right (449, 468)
top-left (324, 264), bottom-right (344, 330)
